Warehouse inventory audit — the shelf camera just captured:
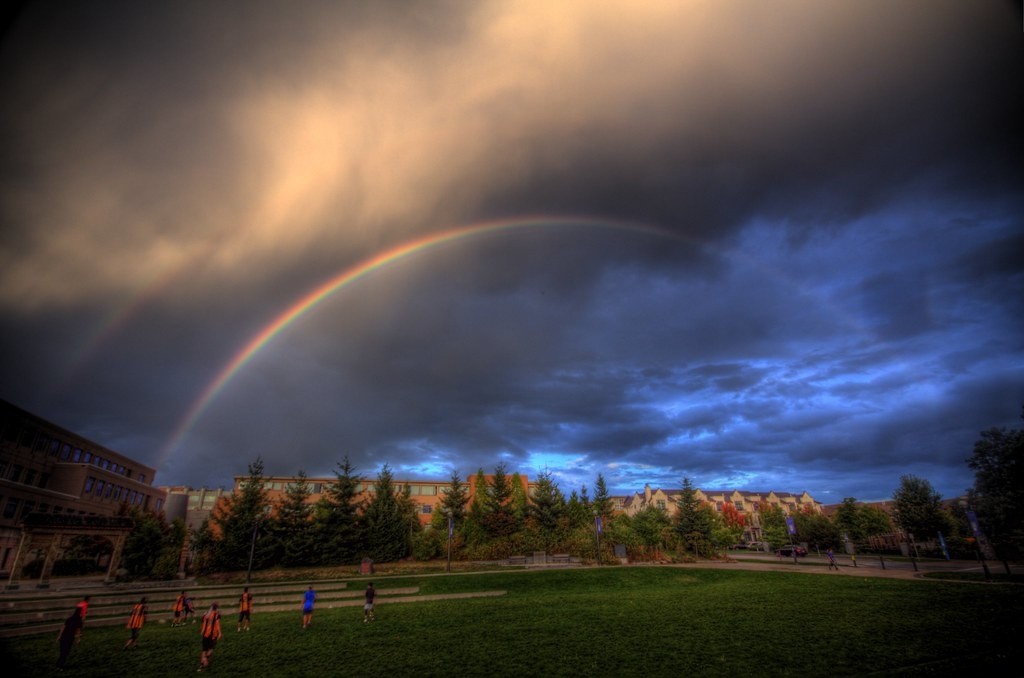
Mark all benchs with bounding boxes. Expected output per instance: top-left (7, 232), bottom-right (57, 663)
top-left (508, 556), bottom-right (525, 564)
top-left (552, 554), bottom-right (570, 563)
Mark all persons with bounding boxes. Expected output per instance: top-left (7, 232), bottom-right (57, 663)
top-left (56, 608), bottom-right (82, 672)
top-left (171, 590), bottom-right (187, 628)
top-left (364, 582), bottom-right (375, 622)
top-left (827, 545), bottom-right (838, 570)
top-left (75, 595), bottom-right (90, 637)
top-left (200, 602), bottom-right (222, 665)
top-left (184, 597), bottom-right (195, 622)
top-left (122, 597), bottom-right (147, 652)
top-left (237, 588), bottom-right (253, 632)
top-left (301, 584), bottom-right (316, 627)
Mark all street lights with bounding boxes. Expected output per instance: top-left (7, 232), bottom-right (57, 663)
top-left (782, 510), bottom-right (798, 563)
top-left (445, 512), bottom-right (455, 572)
top-left (893, 509), bottom-right (918, 571)
top-left (593, 509), bottom-right (603, 568)
top-left (246, 514), bottom-right (263, 583)
top-left (8, 523), bottom-right (28, 586)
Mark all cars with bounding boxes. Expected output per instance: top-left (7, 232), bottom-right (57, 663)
top-left (774, 545), bottom-right (808, 559)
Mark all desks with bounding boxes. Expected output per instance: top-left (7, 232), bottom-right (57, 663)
top-left (532, 551), bottom-right (546, 564)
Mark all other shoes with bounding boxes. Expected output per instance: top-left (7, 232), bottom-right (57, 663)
top-left (171, 622), bottom-right (175, 627)
top-left (197, 668), bottom-right (206, 672)
top-left (191, 620), bottom-right (196, 624)
top-left (175, 623), bottom-right (180, 626)
top-left (182, 623), bottom-right (187, 626)
top-left (201, 659), bottom-right (208, 666)
top-left (364, 620), bottom-right (368, 624)
top-left (369, 615), bottom-right (374, 620)
